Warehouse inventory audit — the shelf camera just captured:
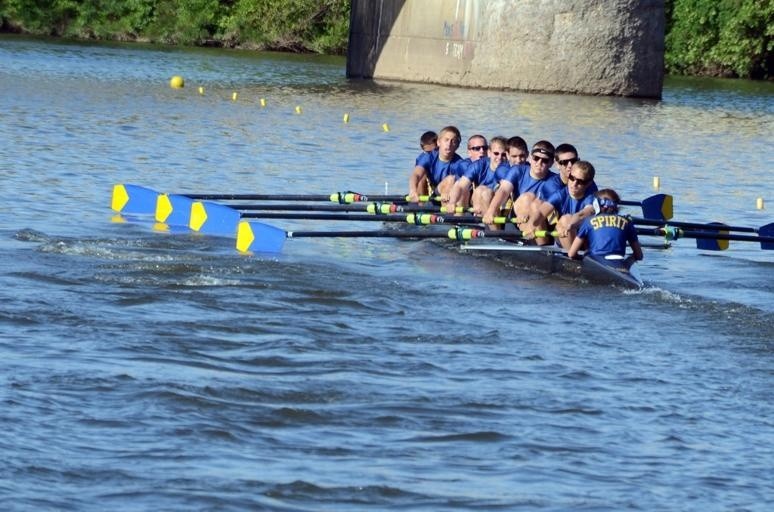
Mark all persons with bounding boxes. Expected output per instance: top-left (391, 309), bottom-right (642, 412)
top-left (408, 126), bottom-right (644, 261)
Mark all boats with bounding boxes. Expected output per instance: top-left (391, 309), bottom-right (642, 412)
top-left (382, 209), bottom-right (646, 292)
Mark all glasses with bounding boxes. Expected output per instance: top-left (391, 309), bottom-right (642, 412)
top-left (469, 145), bottom-right (488, 151)
top-left (531, 154), bottom-right (550, 164)
top-left (492, 152), bottom-right (505, 156)
top-left (559, 157), bottom-right (578, 166)
top-left (568, 175), bottom-right (585, 184)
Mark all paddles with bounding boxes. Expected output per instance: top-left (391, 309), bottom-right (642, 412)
top-left (616, 192), bottom-right (673, 222)
top-left (635, 216), bottom-right (774, 251)
top-left (156, 192), bottom-right (468, 225)
top-left (189, 203), bottom-right (528, 238)
top-left (110, 182), bottom-right (451, 215)
top-left (634, 221), bottom-right (729, 250)
top-left (235, 218), bottom-right (569, 255)
